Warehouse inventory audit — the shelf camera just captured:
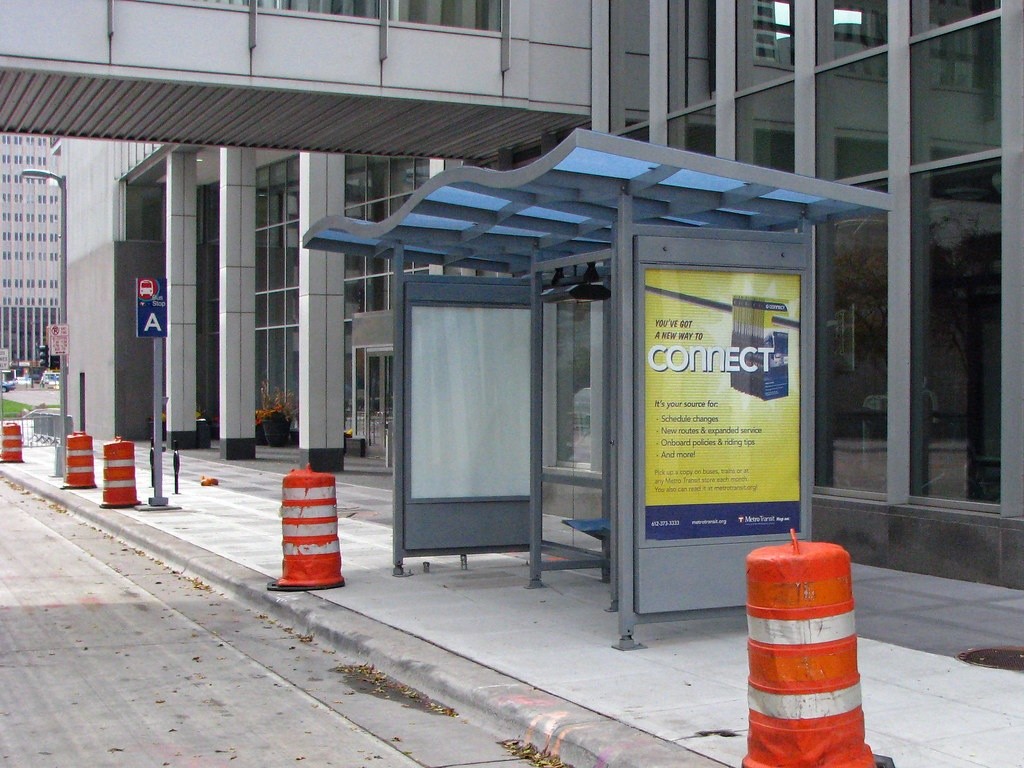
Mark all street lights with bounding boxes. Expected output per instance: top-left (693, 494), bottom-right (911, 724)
top-left (19, 168), bottom-right (68, 479)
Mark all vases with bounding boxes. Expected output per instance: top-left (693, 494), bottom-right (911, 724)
top-left (262, 421), bottom-right (291, 445)
top-left (256, 424), bottom-right (266, 445)
top-left (150, 422), bottom-right (166, 439)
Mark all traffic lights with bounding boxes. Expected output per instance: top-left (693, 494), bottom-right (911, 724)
top-left (39, 344), bottom-right (47, 366)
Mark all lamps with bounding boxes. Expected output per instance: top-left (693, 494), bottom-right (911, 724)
top-left (540, 262), bottom-right (611, 304)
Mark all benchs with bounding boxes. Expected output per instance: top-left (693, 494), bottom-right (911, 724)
top-left (561, 516), bottom-right (609, 543)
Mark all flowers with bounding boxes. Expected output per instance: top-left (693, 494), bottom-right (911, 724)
top-left (344, 429), bottom-right (353, 437)
top-left (255, 381), bottom-right (299, 424)
top-left (147, 412), bottom-right (166, 422)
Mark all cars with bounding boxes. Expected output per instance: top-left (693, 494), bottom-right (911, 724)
top-left (1, 372), bottom-right (59, 393)
